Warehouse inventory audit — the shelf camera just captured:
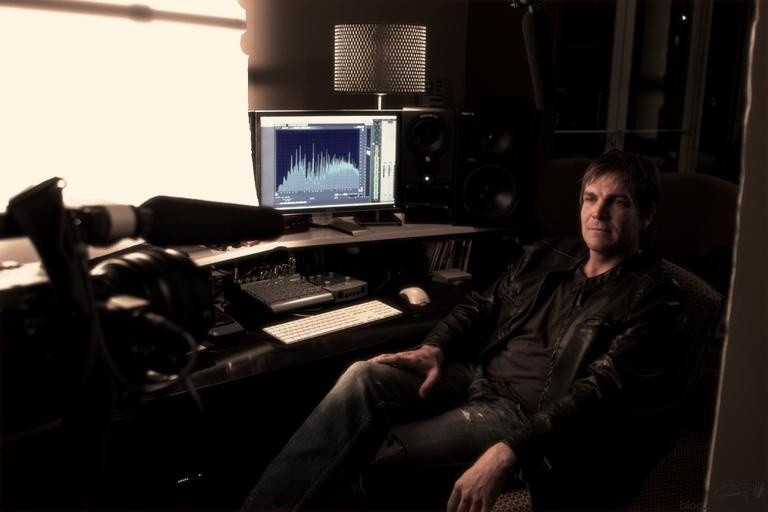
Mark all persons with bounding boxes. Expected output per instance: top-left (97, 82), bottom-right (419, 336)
top-left (240, 150), bottom-right (684, 512)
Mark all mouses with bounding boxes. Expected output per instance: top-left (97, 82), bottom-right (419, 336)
top-left (399, 287), bottom-right (431, 306)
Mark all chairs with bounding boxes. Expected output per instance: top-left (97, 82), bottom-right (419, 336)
top-left (487, 253), bottom-right (724, 512)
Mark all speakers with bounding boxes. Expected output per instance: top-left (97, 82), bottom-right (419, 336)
top-left (401, 107), bottom-right (457, 225)
top-left (451, 97), bottom-right (542, 240)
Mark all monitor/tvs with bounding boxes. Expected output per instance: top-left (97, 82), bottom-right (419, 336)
top-left (249, 109), bottom-right (402, 237)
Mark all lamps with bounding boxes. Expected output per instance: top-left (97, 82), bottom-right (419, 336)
top-left (329, 20), bottom-right (429, 111)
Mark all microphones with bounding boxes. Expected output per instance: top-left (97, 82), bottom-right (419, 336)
top-left (138, 195), bottom-right (284, 249)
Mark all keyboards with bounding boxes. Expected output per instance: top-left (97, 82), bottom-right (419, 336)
top-left (262, 300), bottom-right (403, 345)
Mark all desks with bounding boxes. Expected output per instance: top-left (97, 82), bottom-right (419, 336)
top-left (142, 222), bottom-right (497, 401)
